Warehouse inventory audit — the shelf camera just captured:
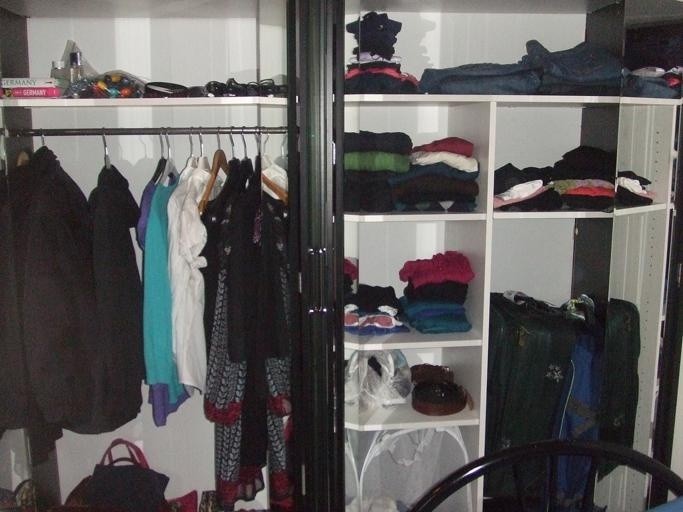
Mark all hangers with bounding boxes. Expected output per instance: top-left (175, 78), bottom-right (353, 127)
top-left (0, 125), bottom-right (289, 232)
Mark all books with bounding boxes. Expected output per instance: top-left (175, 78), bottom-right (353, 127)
top-left (0, 77), bottom-right (70, 88)
top-left (0, 88), bottom-right (69, 98)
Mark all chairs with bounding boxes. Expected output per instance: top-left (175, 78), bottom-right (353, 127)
top-left (412, 438), bottom-right (682, 512)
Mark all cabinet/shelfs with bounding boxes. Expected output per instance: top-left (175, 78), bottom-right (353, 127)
top-left (321, 0), bottom-right (682, 512)
top-left (0, 0), bottom-right (322, 511)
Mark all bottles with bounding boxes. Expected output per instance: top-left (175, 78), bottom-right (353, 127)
top-left (51, 60), bottom-right (65, 78)
